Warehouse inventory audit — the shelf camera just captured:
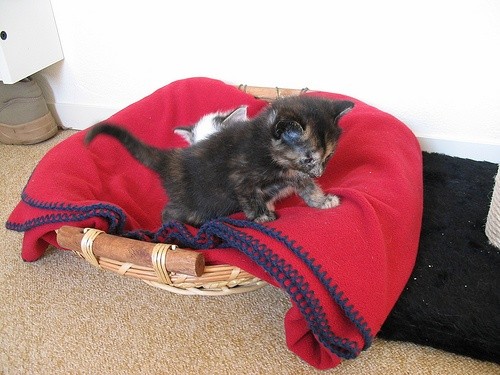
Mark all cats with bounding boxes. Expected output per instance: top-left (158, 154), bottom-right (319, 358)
top-left (84, 94), bottom-right (355, 229)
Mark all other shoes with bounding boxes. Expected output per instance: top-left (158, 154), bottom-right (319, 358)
top-left (0, 76), bottom-right (58, 145)
